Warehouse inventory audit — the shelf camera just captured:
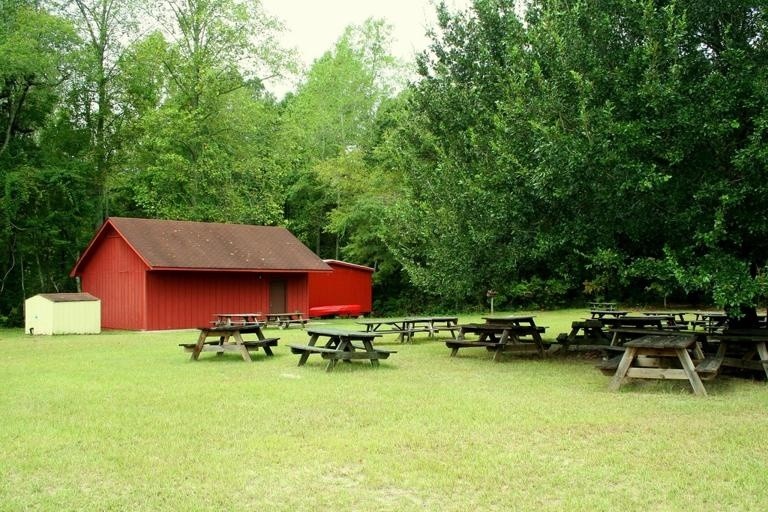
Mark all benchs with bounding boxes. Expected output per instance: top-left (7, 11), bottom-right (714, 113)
top-left (355, 313), bottom-right (464, 347)
top-left (281, 327), bottom-right (398, 372)
top-left (262, 312), bottom-right (310, 331)
top-left (550, 308), bottom-right (768, 372)
top-left (442, 314), bottom-right (554, 360)
top-left (176, 308), bottom-right (281, 369)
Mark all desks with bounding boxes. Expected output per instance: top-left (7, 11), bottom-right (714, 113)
top-left (610, 335), bottom-right (709, 397)
top-left (590, 302), bottom-right (616, 310)
